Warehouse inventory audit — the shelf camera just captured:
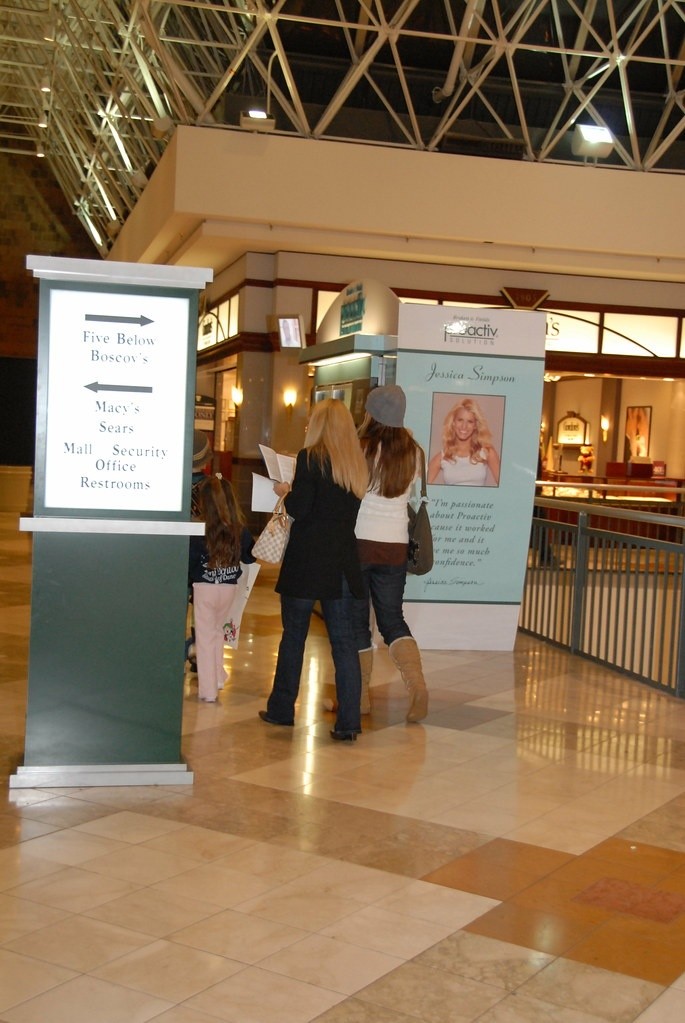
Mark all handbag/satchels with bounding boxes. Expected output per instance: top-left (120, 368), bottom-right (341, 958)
top-left (251, 493), bottom-right (295, 563)
top-left (406, 446), bottom-right (434, 576)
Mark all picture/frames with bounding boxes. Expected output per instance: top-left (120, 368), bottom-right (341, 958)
top-left (625, 405), bottom-right (653, 457)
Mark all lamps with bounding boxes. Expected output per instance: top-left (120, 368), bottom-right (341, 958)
top-left (39, 75), bottom-right (51, 93)
top-left (239, 110), bottom-right (277, 130)
top-left (36, 145), bottom-right (44, 158)
top-left (43, 24), bottom-right (56, 42)
top-left (572, 123), bottom-right (614, 166)
top-left (38, 110), bottom-right (48, 129)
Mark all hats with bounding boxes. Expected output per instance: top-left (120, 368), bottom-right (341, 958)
top-left (364, 384), bottom-right (407, 427)
top-left (192, 430), bottom-right (210, 461)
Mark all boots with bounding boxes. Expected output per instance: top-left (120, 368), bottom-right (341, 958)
top-left (387, 636), bottom-right (429, 722)
top-left (322, 647), bottom-right (374, 714)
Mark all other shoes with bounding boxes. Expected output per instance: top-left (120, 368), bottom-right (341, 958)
top-left (217, 682), bottom-right (224, 689)
top-left (329, 729), bottom-right (358, 741)
top-left (258, 710), bottom-right (294, 726)
top-left (204, 696), bottom-right (216, 702)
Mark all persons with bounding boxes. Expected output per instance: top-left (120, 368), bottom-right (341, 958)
top-left (534, 448), bottom-right (566, 566)
top-left (259, 399), bottom-right (371, 742)
top-left (322, 386), bottom-right (429, 723)
top-left (427, 398), bottom-right (501, 486)
top-left (182, 428), bottom-right (256, 704)
